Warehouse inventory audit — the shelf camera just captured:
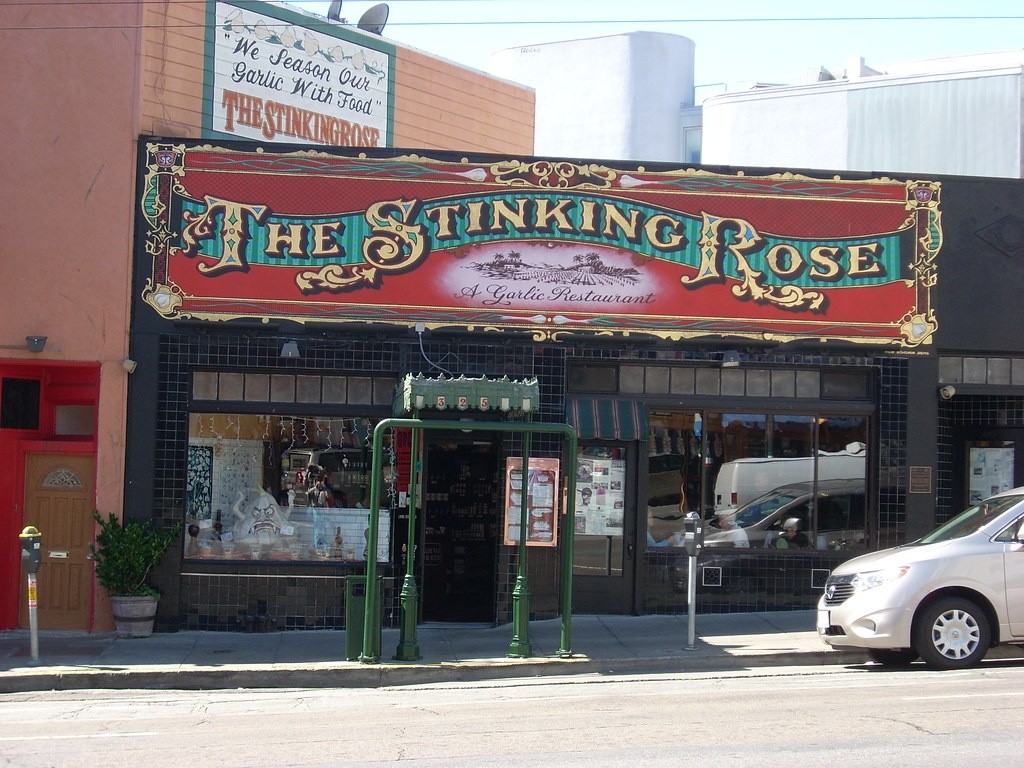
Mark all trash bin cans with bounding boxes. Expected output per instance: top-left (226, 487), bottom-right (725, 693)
top-left (344, 575), bottom-right (384, 661)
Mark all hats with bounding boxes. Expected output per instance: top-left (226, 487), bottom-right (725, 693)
top-left (715, 508), bottom-right (738, 519)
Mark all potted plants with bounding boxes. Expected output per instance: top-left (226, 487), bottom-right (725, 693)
top-left (87, 510), bottom-right (184, 639)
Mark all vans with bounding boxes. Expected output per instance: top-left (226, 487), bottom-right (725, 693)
top-left (283, 446), bottom-right (394, 508)
top-left (713, 452), bottom-right (879, 524)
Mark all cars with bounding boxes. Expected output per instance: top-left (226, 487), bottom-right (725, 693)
top-left (816, 485), bottom-right (1024, 670)
top-left (701, 477), bottom-right (868, 549)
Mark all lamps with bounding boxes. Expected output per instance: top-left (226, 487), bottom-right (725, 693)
top-left (280, 342), bottom-right (300, 359)
top-left (121, 359), bottom-right (136, 374)
top-left (722, 350), bottom-right (740, 368)
top-left (940, 385), bottom-right (956, 400)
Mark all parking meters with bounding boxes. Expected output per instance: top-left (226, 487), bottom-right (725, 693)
top-left (684, 511), bottom-right (703, 651)
top-left (18, 526), bottom-right (49, 666)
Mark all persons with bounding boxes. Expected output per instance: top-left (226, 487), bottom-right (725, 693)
top-left (577, 487), bottom-right (592, 506)
top-left (306, 481), bottom-right (331, 558)
top-left (647, 510), bottom-right (675, 547)
top-left (703, 510), bottom-right (750, 548)
top-left (775, 516), bottom-right (810, 548)
